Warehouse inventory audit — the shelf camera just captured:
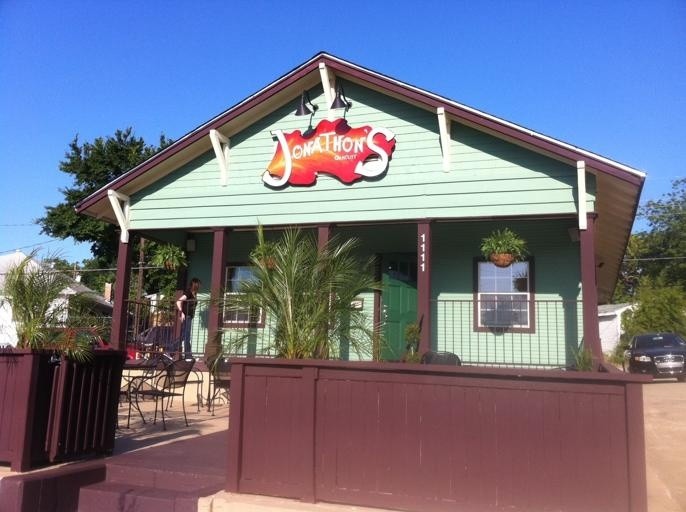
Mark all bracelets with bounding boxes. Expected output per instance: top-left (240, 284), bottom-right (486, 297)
top-left (178, 310), bottom-right (184, 315)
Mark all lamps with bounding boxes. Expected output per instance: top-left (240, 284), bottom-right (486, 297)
top-left (295, 79), bottom-right (350, 117)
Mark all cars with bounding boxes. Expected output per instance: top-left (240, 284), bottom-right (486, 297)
top-left (621, 332), bottom-right (686, 382)
top-left (18, 327), bottom-right (159, 370)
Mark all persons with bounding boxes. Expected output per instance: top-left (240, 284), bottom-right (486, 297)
top-left (169, 276), bottom-right (202, 362)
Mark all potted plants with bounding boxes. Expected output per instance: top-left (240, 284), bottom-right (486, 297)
top-left (146, 241), bottom-right (190, 270)
top-left (479, 226), bottom-right (533, 267)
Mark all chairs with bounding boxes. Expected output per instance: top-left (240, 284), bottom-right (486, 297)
top-left (419, 349), bottom-right (463, 366)
top-left (115, 351), bottom-right (238, 429)
